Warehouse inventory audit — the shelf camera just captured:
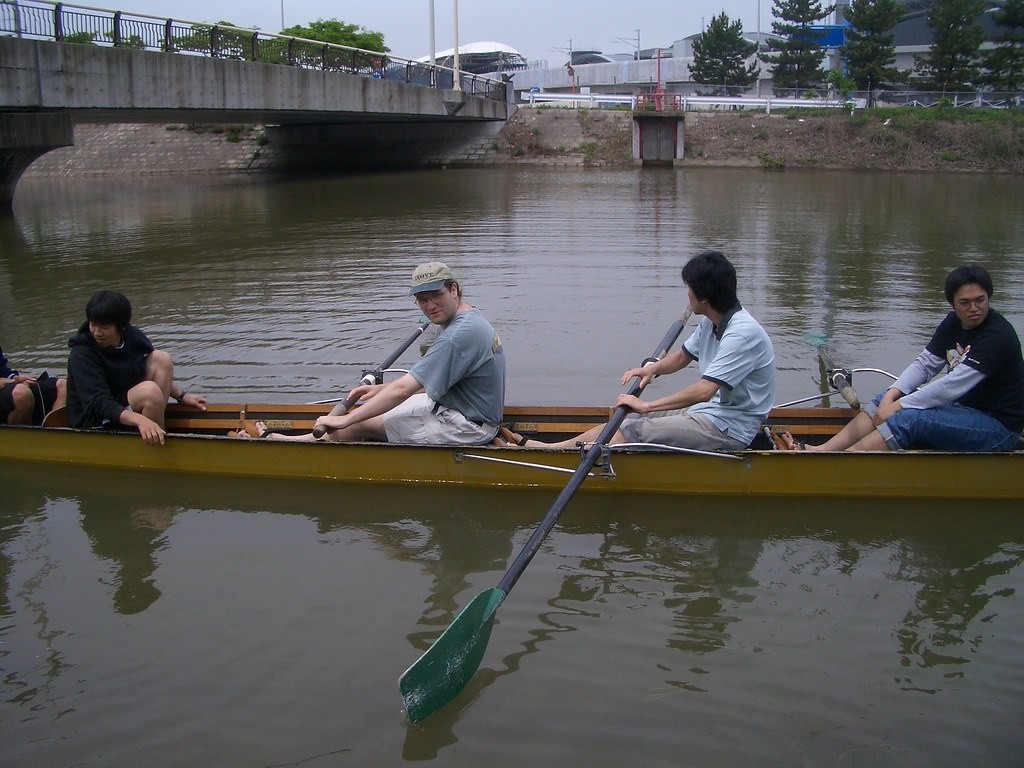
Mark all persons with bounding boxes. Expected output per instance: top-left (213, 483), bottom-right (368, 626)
top-left (507, 249), bottom-right (777, 451)
top-left (64, 290), bottom-right (207, 445)
top-left (0, 341), bottom-right (68, 426)
top-left (782, 266), bottom-right (1024, 453)
top-left (238, 261), bottom-right (506, 446)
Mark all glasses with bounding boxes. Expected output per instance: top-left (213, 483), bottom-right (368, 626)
top-left (414, 287), bottom-right (449, 306)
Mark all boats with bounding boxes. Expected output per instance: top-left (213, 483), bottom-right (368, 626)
top-left (1, 404), bottom-right (1023, 500)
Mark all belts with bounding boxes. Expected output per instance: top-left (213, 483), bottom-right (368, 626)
top-left (470, 419), bottom-right (484, 427)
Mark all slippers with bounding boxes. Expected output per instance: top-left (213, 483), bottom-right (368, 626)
top-left (500, 426), bottom-right (530, 446)
top-left (769, 429), bottom-right (789, 449)
top-left (227, 430), bottom-right (249, 438)
top-left (774, 439), bottom-right (807, 451)
top-left (493, 437), bottom-right (510, 447)
top-left (243, 421), bottom-right (272, 438)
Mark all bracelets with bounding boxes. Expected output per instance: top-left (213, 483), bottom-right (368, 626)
top-left (177, 390), bottom-right (187, 403)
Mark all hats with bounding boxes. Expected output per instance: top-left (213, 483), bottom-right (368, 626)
top-left (408, 262), bottom-right (453, 297)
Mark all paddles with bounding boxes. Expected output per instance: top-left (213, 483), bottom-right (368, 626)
top-left (312, 314), bottom-right (432, 438)
top-left (397, 303), bottom-right (694, 724)
top-left (817, 348), bottom-right (859, 410)
